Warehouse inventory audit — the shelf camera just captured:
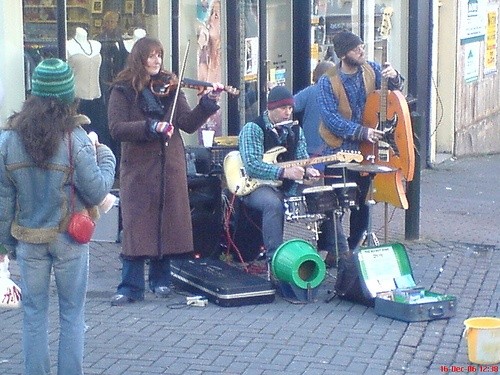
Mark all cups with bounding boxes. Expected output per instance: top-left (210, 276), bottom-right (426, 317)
top-left (202, 130), bottom-right (215, 147)
top-left (393, 288), bottom-right (409, 303)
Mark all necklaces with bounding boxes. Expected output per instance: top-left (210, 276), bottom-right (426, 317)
top-left (73, 37), bottom-right (93, 56)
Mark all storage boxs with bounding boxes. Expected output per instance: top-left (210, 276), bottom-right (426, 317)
top-left (354, 243), bottom-right (457, 322)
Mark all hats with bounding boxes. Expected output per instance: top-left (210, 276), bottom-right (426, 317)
top-left (30, 57), bottom-right (75, 101)
top-left (332, 29), bottom-right (364, 58)
top-left (267, 86), bottom-right (294, 110)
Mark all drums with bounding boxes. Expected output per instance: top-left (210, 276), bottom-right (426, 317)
top-left (301, 186), bottom-right (338, 214)
top-left (331, 182), bottom-right (361, 208)
top-left (284, 195), bottom-right (327, 223)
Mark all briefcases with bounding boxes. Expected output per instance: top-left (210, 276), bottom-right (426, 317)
top-left (352, 240), bottom-right (457, 322)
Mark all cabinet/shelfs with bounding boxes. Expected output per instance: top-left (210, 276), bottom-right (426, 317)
top-left (23, 0), bottom-right (91, 42)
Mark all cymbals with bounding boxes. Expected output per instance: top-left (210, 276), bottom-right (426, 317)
top-left (326, 162), bottom-right (397, 173)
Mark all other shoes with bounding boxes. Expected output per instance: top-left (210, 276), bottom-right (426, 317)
top-left (324, 252), bottom-right (354, 269)
top-left (153, 285), bottom-right (171, 298)
top-left (111, 294), bottom-right (131, 306)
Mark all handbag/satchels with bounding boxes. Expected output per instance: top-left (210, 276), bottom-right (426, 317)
top-left (66, 212), bottom-right (96, 244)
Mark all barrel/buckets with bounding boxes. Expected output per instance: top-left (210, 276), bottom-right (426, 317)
top-left (463, 317), bottom-right (500, 364)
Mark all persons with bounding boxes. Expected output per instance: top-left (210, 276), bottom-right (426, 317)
top-left (107, 38), bottom-right (224, 307)
top-left (66, 27), bottom-right (111, 148)
top-left (196, 1), bottom-right (222, 140)
top-left (237, 86), bottom-right (349, 268)
top-left (0, 58), bottom-right (116, 375)
top-left (317, 29), bottom-right (404, 251)
top-left (108, 28), bottom-right (146, 77)
top-left (292, 61), bottom-right (336, 173)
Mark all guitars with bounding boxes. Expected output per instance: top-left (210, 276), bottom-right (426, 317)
top-left (223, 146), bottom-right (364, 196)
top-left (360, 6), bottom-right (416, 209)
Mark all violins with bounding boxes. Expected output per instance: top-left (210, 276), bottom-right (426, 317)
top-left (150, 70), bottom-right (240, 96)
